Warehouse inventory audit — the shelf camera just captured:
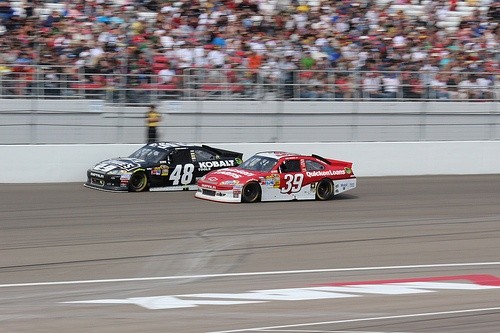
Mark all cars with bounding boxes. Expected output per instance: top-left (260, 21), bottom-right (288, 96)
top-left (82, 142), bottom-right (245, 193)
top-left (195, 150), bottom-right (359, 203)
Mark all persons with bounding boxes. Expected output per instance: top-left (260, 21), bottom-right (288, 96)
top-left (145, 104), bottom-right (160, 145)
top-left (0, 0), bottom-right (500, 102)
